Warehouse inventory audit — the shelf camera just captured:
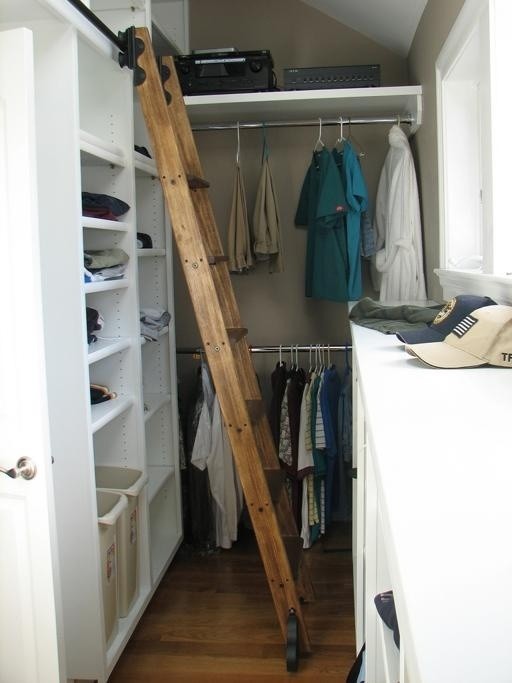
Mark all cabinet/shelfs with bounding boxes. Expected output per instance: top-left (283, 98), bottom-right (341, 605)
top-left (343, 296), bottom-right (512, 677)
top-left (0, 0), bottom-right (191, 683)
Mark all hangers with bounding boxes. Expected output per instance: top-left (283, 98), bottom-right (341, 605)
top-left (232, 120), bottom-right (272, 167)
top-left (313, 113), bottom-right (367, 161)
top-left (277, 342), bottom-right (353, 385)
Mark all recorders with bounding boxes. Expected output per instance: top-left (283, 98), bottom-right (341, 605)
top-left (173, 49), bottom-right (274, 96)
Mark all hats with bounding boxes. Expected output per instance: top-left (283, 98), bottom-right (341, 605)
top-left (395, 294), bottom-right (512, 368)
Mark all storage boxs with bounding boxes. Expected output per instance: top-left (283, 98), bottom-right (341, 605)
top-left (90, 463), bottom-right (154, 654)
